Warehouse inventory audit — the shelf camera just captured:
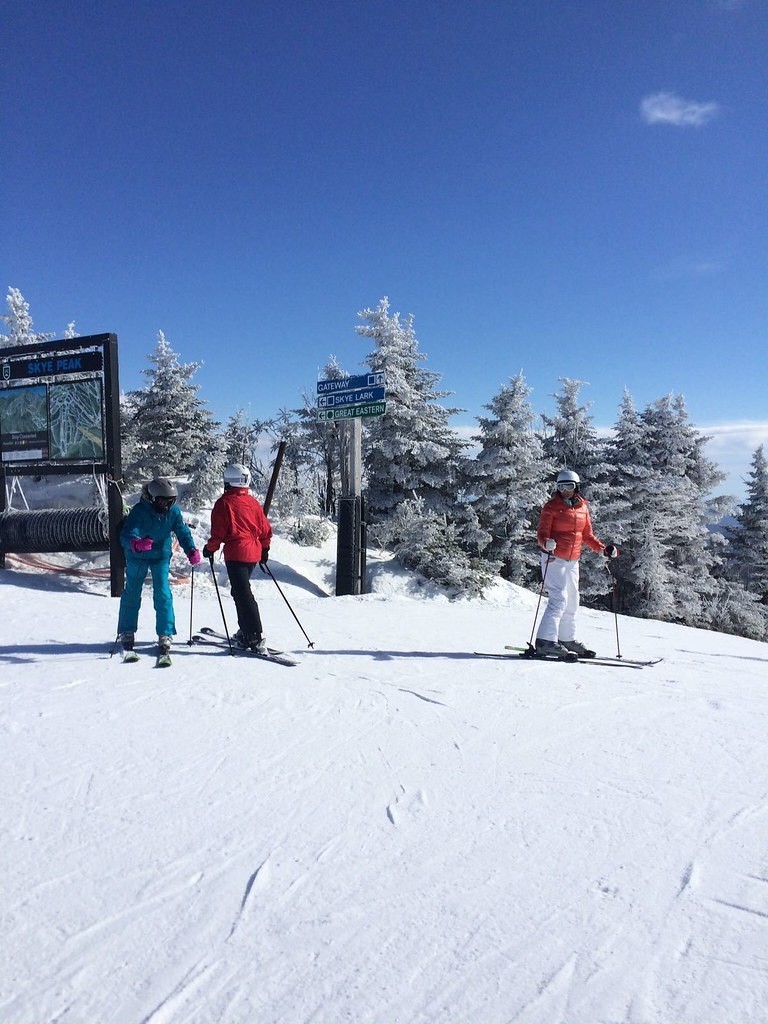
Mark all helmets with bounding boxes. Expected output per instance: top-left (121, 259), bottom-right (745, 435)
top-left (224, 464), bottom-right (251, 488)
top-left (148, 478), bottom-right (179, 501)
top-left (557, 470), bottom-right (581, 484)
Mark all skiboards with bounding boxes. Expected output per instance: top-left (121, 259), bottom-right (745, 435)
top-left (192, 628), bottom-right (302, 666)
top-left (119, 631), bottom-right (173, 668)
top-left (474, 636), bottom-right (665, 669)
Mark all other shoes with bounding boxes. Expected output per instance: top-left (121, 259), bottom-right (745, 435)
top-left (120, 631), bottom-right (134, 651)
top-left (158, 634), bottom-right (173, 653)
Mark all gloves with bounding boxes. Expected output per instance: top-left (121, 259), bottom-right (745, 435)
top-left (186, 549), bottom-right (200, 566)
top-left (260, 547), bottom-right (270, 564)
top-left (130, 536), bottom-right (153, 553)
top-left (544, 539), bottom-right (557, 551)
top-left (203, 545), bottom-right (214, 557)
top-left (604, 545), bottom-right (620, 559)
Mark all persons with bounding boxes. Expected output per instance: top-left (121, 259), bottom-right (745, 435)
top-left (203, 464), bottom-right (272, 656)
top-left (116, 478), bottom-right (200, 657)
top-left (537, 471), bottom-right (619, 661)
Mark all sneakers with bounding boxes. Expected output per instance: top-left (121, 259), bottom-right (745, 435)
top-left (228, 628), bottom-right (244, 644)
top-left (535, 639), bottom-right (578, 660)
top-left (558, 639), bottom-right (596, 658)
top-left (234, 632), bottom-right (262, 650)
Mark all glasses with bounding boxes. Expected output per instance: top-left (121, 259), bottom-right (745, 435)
top-left (558, 482), bottom-right (576, 492)
